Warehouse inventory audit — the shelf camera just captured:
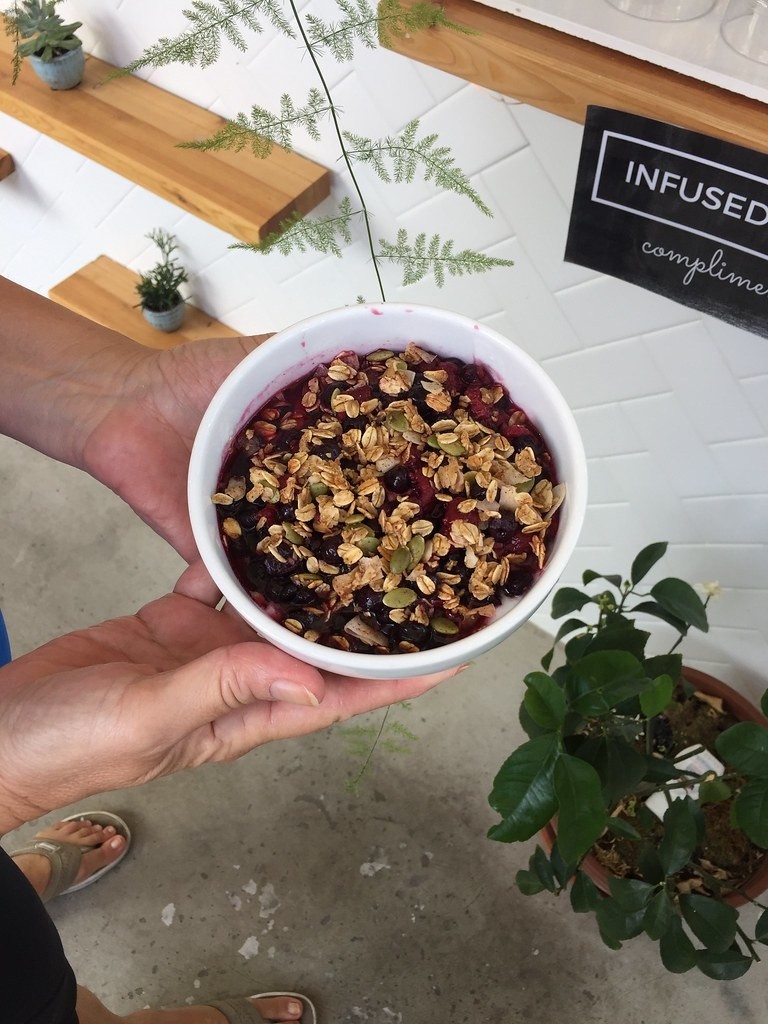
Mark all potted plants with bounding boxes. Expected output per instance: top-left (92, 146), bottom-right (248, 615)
top-left (133, 228), bottom-right (190, 331)
top-left (486, 541), bottom-right (768, 981)
top-left (4, 0), bottom-right (85, 90)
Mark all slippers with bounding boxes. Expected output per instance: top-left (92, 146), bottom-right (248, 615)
top-left (7, 811), bottom-right (131, 903)
top-left (201, 992), bottom-right (316, 1024)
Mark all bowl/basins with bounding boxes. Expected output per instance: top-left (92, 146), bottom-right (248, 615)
top-left (187, 305), bottom-right (587, 680)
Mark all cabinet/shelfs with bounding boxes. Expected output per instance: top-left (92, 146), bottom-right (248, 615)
top-left (0, 1), bottom-right (768, 351)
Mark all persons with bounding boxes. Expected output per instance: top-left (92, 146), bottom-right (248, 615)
top-left (0, 274), bottom-right (475, 1024)
